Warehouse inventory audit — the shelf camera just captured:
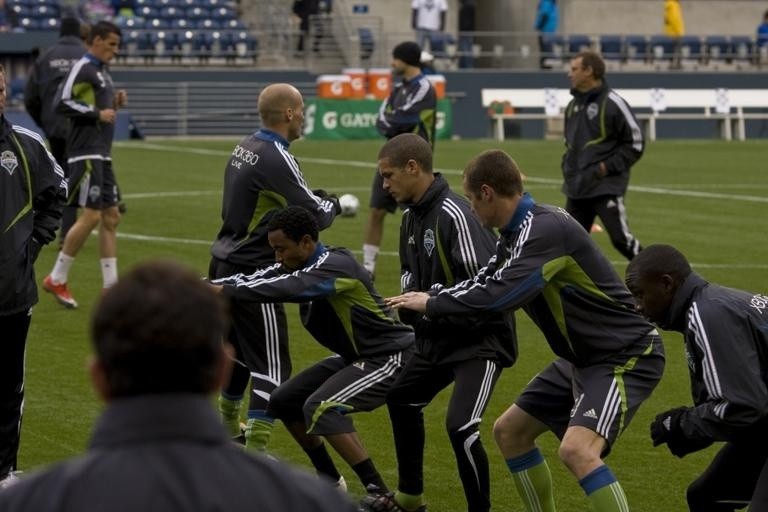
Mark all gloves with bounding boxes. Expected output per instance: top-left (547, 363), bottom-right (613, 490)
top-left (651, 410), bottom-right (670, 447)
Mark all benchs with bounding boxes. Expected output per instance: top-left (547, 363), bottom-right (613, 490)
top-left (479, 86), bottom-right (768, 141)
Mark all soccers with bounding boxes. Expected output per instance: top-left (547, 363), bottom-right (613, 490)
top-left (339, 194), bottom-right (359, 216)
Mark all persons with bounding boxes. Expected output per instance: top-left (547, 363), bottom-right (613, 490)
top-left (292, 0), bottom-right (331, 57)
top-left (624, 243), bottom-right (768, 511)
top-left (410, 0), bottom-right (448, 50)
top-left (1, 258), bottom-right (368, 512)
top-left (534, 0), bottom-right (560, 69)
top-left (1, 64), bottom-right (70, 484)
top-left (660, 0), bottom-right (687, 37)
top-left (24, 18), bottom-right (89, 249)
top-left (1, 0), bottom-right (138, 32)
top-left (202, 206), bottom-right (414, 495)
top-left (755, 10), bottom-right (768, 56)
top-left (203, 84), bottom-right (343, 463)
top-left (560, 51), bottom-right (646, 264)
top-left (362, 131), bottom-right (519, 511)
top-left (42, 19), bottom-right (127, 309)
top-left (362, 41), bottom-right (437, 275)
top-left (384, 149), bottom-right (664, 511)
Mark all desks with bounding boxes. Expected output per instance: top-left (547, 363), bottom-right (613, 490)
top-left (303, 99), bottom-right (453, 143)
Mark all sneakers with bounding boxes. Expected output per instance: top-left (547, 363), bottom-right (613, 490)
top-left (330, 476), bottom-right (425, 512)
top-left (43, 276), bottom-right (78, 308)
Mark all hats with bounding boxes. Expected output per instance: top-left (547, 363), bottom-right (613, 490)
top-left (393, 42), bottom-right (420, 65)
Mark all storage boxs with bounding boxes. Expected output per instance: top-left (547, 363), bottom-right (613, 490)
top-left (316, 75), bottom-right (352, 100)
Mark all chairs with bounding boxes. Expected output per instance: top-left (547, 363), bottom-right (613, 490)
top-left (542, 34), bottom-right (768, 72)
top-left (0, 0), bottom-right (260, 66)
